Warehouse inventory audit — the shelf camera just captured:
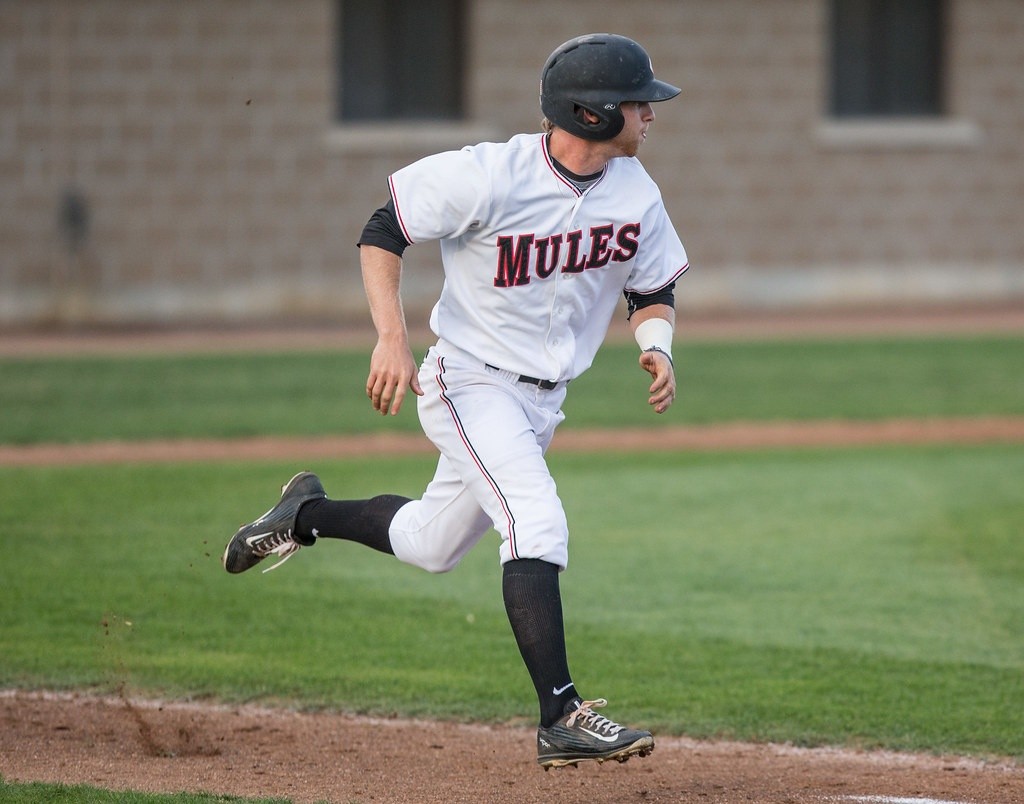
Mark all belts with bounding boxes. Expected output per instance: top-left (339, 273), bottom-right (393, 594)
top-left (485, 363), bottom-right (557, 390)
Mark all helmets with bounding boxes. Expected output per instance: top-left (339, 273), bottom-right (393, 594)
top-left (539, 33), bottom-right (682, 140)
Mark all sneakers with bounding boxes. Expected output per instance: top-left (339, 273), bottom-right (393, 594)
top-left (223, 471), bottom-right (327, 574)
top-left (536, 696), bottom-right (654, 770)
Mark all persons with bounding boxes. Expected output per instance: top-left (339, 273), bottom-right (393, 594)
top-left (219, 32), bottom-right (690, 772)
